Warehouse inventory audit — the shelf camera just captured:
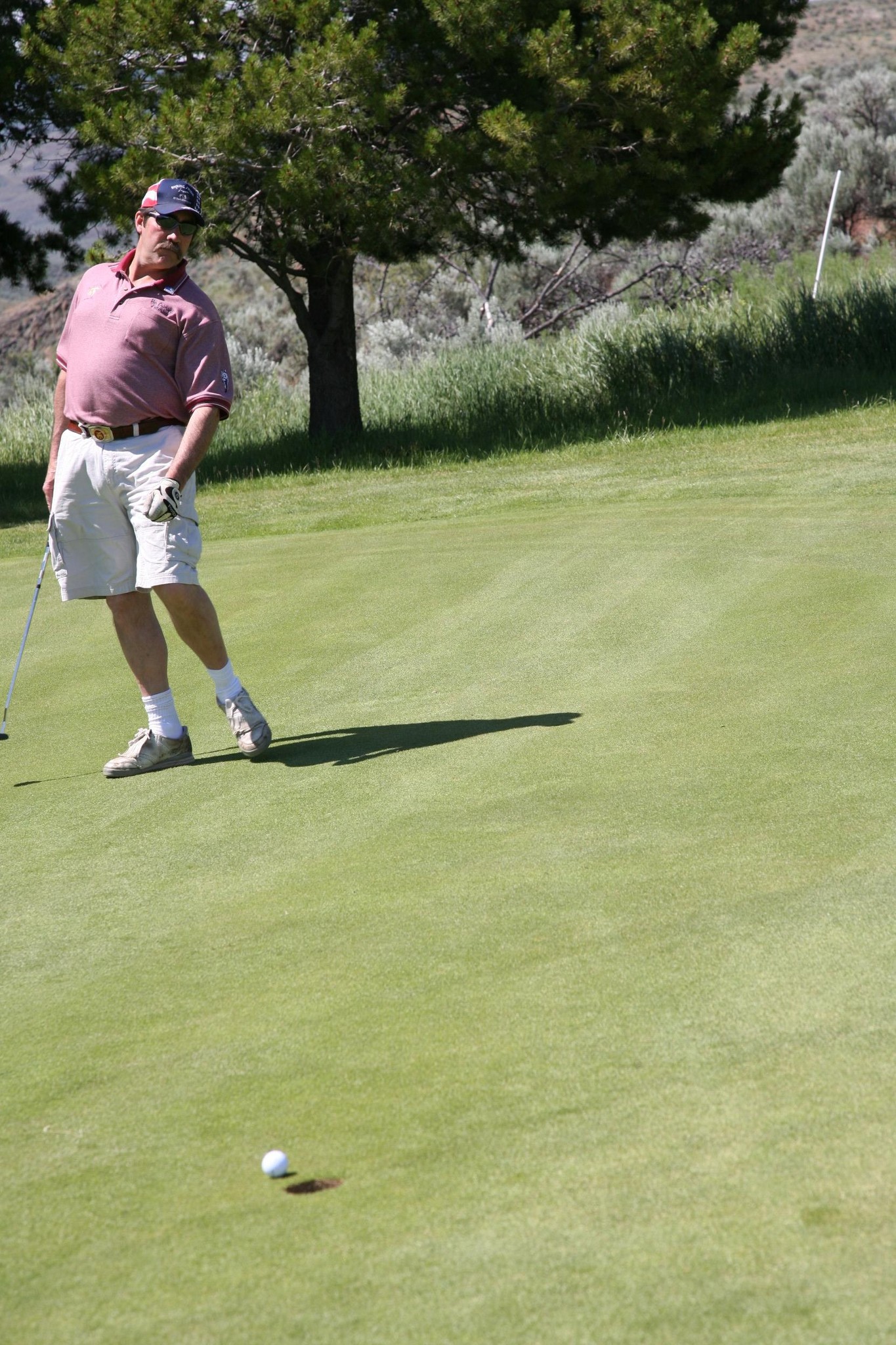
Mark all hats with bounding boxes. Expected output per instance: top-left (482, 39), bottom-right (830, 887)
top-left (141, 178), bottom-right (205, 227)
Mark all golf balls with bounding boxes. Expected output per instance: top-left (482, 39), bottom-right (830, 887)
top-left (261, 1150), bottom-right (288, 1178)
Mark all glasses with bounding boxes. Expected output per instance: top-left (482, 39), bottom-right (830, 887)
top-left (148, 212), bottom-right (197, 236)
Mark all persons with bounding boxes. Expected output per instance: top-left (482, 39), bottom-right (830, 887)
top-left (43, 178), bottom-right (273, 778)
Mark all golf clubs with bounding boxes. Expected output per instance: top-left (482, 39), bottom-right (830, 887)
top-left (0, 540), bottom-right (50, 742)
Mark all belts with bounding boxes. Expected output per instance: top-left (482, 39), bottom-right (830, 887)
top-left (66, 417), bottom-right (187, 443)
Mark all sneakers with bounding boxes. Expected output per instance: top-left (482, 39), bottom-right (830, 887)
top-left (103, 726), bottom-right (195, 776)
top-left (216, 687), bottom-right (272, 757)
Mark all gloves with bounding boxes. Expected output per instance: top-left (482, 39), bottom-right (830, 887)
top-left (143, 477), bottom-right (183, 522)
